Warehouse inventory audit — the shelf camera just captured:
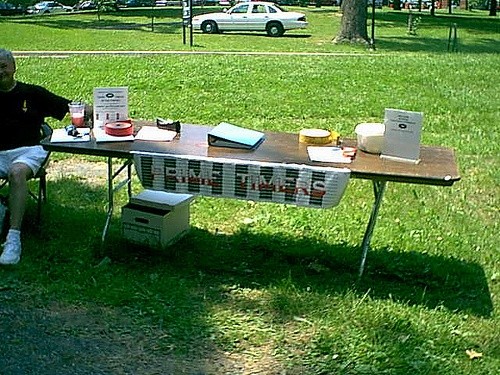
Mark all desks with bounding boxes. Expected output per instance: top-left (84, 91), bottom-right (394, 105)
top-left (39, 120), bottom-right (459, 276)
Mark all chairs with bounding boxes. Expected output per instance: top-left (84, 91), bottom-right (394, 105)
top-left (1, 122), bottom-right (51, 205)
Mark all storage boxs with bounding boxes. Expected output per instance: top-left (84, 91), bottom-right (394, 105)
top-left (122, 190), bottom-right (194, 253)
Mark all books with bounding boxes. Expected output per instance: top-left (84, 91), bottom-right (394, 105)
top-left (207, 121), bottom-right (267, 151)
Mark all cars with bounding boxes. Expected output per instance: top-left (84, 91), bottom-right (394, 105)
top-left (73, 0), bottom-right (97, 11)
top-left (123, 0), bottom-right (156, 8)
top-left (0, 1), bottom-right (74, 15)
top-left (191, 1), bottom-right (309, 37)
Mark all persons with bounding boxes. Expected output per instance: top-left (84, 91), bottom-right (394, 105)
top-left (0, 47), bottom-right (94, 264)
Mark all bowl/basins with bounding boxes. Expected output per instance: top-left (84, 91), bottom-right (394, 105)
top-left (354, 123), bottom-right (385, 154)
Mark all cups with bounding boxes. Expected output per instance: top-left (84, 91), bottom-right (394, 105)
top-left (68, 102), bottom-right (85, 127)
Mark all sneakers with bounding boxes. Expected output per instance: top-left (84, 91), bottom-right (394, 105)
top-left (0, 230), bottom-right (23, 265)
